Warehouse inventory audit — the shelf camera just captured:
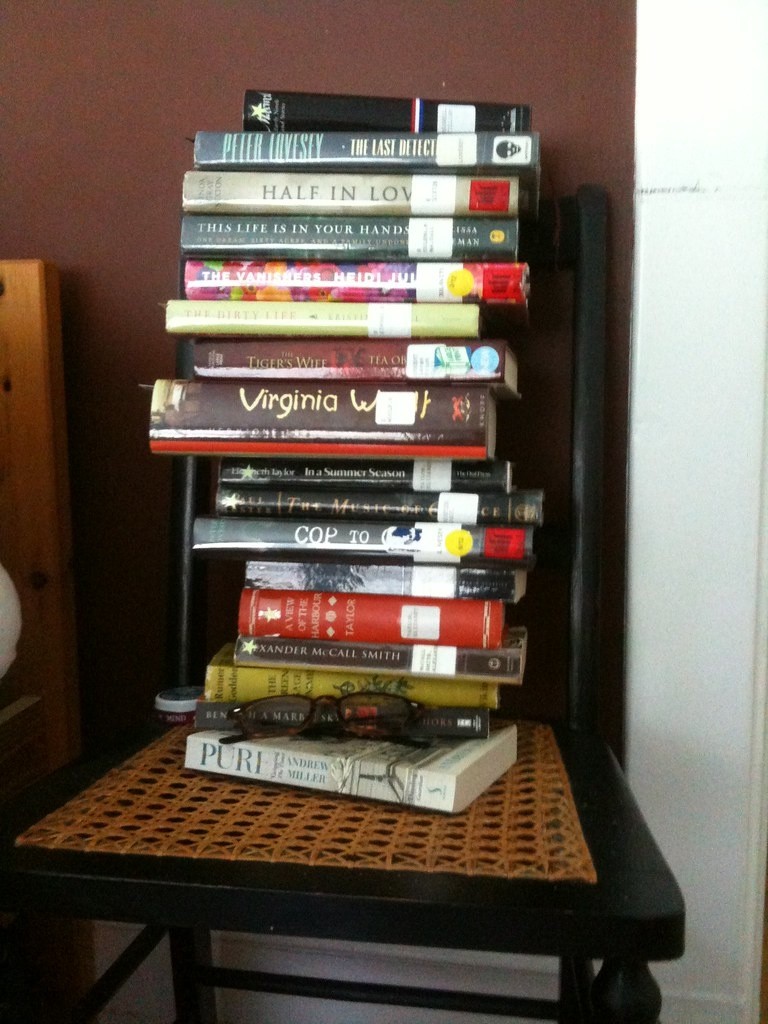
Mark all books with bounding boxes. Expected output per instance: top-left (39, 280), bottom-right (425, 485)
top-left (148, 89), bottom-right (544, 813)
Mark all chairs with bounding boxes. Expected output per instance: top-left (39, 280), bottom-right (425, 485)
top-left (1, 182), bottom-right (687, 1024)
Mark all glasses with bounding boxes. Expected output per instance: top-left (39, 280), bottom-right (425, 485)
top-left (218, 690), bottom-right (432, 749)
